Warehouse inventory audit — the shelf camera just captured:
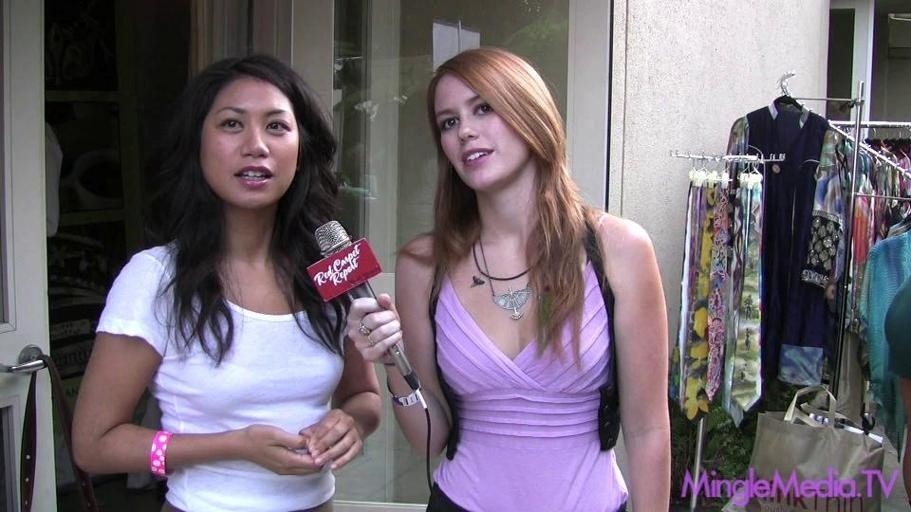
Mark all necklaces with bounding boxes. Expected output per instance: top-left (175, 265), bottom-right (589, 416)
top-left (472, 240), bottom-right (534, 281)
top-left (476, 235), bottom-right (530, 321)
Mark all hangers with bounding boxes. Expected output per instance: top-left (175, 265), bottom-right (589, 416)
top-left (733, 73), bottom-right (911, 256)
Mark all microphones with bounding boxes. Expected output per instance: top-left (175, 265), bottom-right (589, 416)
top-left (306, 219), bottom-right (422, 396)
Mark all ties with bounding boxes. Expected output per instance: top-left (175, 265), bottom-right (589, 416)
top-left (668, 182), bottom-right (761, 427)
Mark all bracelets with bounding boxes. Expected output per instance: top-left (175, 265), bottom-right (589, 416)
top-left (150, 430), bottom-right (177, 478)
top-left (391, 391), bottom-right (420, 407)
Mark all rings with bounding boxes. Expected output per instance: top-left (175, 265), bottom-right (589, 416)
top-left (359, 322), bottom-right (371, 336)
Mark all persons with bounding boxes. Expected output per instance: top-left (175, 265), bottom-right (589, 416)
top-left (345, 46), bottom-right (673, 512)
top-left (69, 54), bottom-right (382, 512)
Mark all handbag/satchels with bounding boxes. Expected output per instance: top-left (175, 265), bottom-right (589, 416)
top-left (747, 386), bottom-right (884, 512)
